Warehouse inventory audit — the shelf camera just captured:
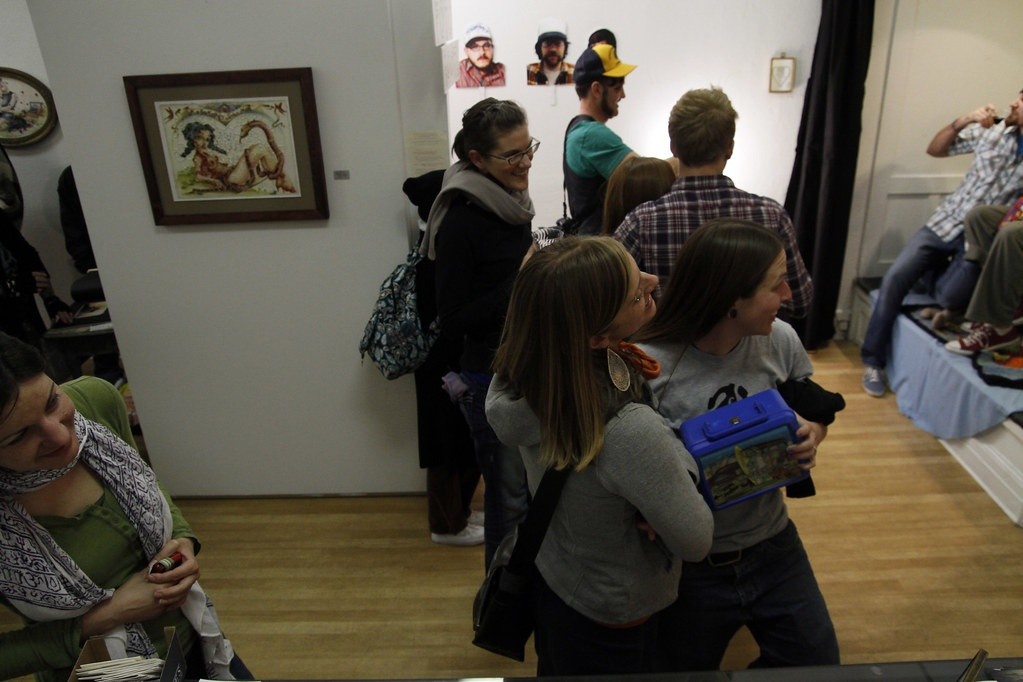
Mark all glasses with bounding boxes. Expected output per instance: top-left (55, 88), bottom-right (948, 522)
top-left (482, 136), bottom-right (540, 167)
top-left (544, 39), bottom-right (565, 48)
top-left (470, 43), bottom-right (493, 52)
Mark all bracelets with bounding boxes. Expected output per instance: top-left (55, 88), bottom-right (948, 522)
top-left (952, 119), bottom-right (960, 132)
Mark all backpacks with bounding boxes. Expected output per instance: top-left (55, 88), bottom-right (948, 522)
top-left (356, 232), bottom-right (440, 380)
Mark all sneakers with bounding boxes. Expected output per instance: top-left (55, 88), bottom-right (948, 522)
top-left (945, 325), bottom-right (1019, 356)
top-left (862, 365), bottom-right (885, 397)
top-left (465, 509), bottom-right (485, 525)
top-left (961, 306), bottom-right (1023, 333)
top-left (431, 526), bottom-right (485, 546)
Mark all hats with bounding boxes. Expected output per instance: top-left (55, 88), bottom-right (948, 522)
top-left (573, 45), bottom-right (636, 85)
top-left (537, 19), bottom-right (567, 40)
top-left (464, 25), bottom-right (492, 46)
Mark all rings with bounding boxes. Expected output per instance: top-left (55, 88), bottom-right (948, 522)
top-left (812, 446), bottom-right (817, 453)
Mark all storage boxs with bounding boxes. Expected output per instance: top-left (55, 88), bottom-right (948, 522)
top-left (679, 390), bottom-right (814, 506)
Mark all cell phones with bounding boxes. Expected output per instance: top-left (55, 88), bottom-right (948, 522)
top-left (150, 553), bottom-right (184, 575)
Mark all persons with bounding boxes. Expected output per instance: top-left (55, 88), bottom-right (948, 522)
top-left (56, 165), bottom-right (98, 276)
top-left (403, 168), bottom-right (445, 251)
top-left (0, 333), bottom-right (257, 682)
top-left (615, 85), bottom-right (814, 326)
top-left (860, 89), bottom-right (1023, 397)
top-left (562, 43), bottom-right (681, 236)
top-left (413, 97), bottom-right (534, 548)
top-left (587, 29), bottom-right (618, 58)
top-left (0, 173), bottom-right (74, 384)
top-left (603, 156), bottom-right (676, 234)
top-left (456, 23), bottom-right (506, 88)
top-left (626, 217), bottom-right (841, 675)
top-left (527, 22), bottom-right (576, 85)
top-left (484, 233), bottom-right (715, 676)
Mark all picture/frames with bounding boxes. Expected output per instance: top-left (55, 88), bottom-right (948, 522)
top-left (122, 68), bottom-right (331, 227)
top-left (770, 57), bottom-right (796, 93)
top-left (0, 67), bottom-right (56, 147)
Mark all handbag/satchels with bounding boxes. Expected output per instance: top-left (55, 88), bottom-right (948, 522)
top-left (471, 527), bottom-right (548, 662)
